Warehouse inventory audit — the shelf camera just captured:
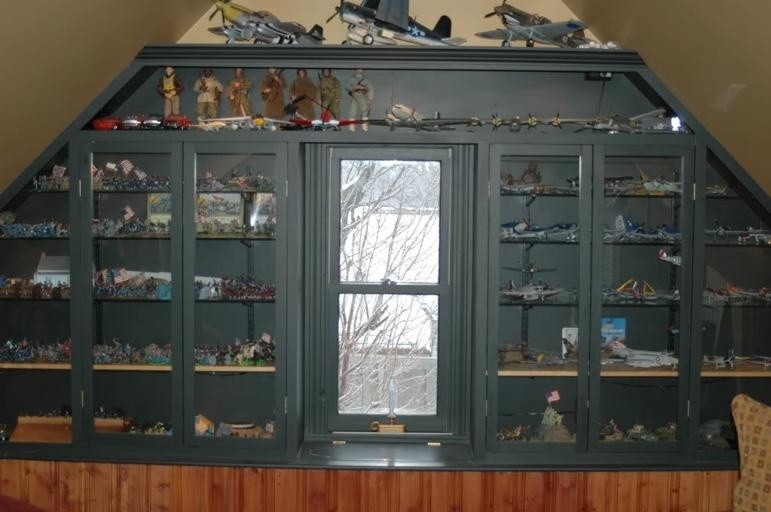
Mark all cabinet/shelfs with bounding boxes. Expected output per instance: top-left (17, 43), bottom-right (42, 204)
top-left (2, 141), bottom-right (288, 458)
top-left (490, 143), bottom-right (769, 454)
top-left (95, 45), bottom-right (723, 143)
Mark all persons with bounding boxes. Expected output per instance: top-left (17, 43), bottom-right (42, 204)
top-left (158, 65), bottom-right (184, 117)
top-left (193, 67), bottom-right (223, 119)
top-left (225, 69), bottom-right (253, 114)
top-left (313, 68), bottom-right (341, 124)
top-left (345, 70), bottom-right (375, 120)
top-left (258, 68), bottom-right (288, 121)
top-left (287, 69), bottom-right (317, 117)
